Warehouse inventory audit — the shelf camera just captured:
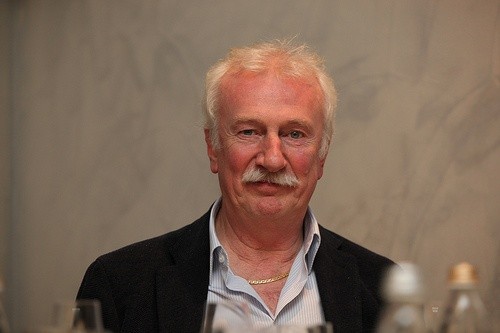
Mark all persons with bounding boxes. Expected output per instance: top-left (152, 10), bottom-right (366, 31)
top-left (72, 35), bottom-right (425, 332)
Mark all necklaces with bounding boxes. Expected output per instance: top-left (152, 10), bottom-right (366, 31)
top-left (247, 273), bottom-right (289, 285)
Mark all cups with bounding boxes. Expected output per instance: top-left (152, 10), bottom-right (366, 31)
top-left (48, 300), bottom-right (103, 333)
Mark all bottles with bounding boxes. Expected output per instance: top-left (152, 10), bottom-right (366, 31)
top-left (371, 260), bottom-right (495, 333)
